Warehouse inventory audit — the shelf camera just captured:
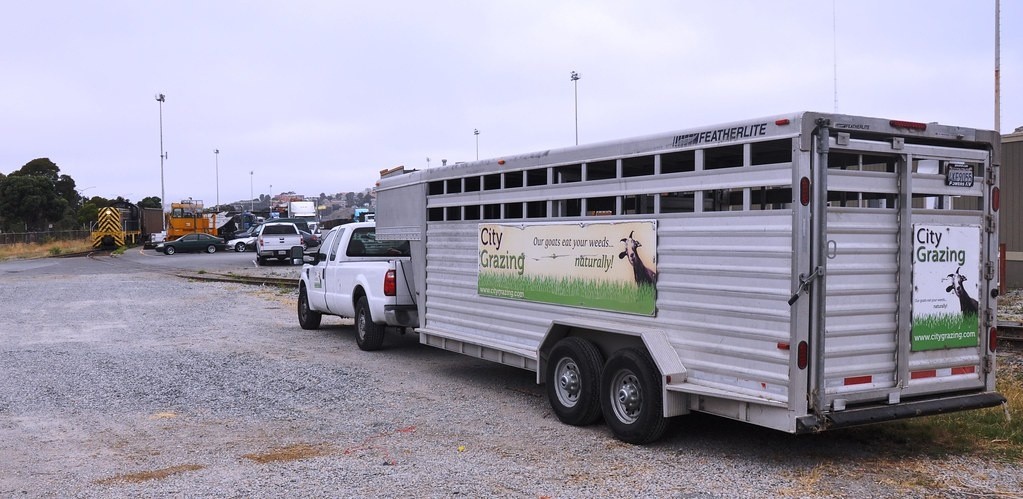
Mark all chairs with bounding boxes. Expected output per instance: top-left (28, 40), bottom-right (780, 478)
top-left (347, 239), bottom-right (367, 257)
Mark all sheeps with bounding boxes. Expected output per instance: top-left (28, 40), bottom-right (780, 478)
top-left (945, 266), bottom-right (978, 317)
top-left (618, 231), bottom-right (656, 285)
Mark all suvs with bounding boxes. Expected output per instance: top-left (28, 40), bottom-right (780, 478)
top-left (235, 218), bottom-right (309, 239)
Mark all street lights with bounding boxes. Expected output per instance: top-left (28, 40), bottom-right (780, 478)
top-left (571, 70), bottom-right (579, 145)
top-left (155, 92), bottom-right (167, 231)
top-left (474, 128), bottom-right (479, 160)
top-left (214, 149), bottom-right (218, 211)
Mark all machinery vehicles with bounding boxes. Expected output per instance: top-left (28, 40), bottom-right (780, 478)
top-left (166, 197), bottom-right (218, 241)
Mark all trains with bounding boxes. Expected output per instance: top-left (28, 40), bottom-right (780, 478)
top-left (90, 200), bottom-right (163, 251)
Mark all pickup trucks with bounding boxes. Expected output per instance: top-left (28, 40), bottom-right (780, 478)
top-left (251, 223), bottom-right (304, 265)
top-left (296, 221), bottom-right (419, 352)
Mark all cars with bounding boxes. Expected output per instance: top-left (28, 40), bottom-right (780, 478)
top-left (299, 229), bottom-right (318, 251)
top-left (226, 237), bottom-right (259, 252)
top-left (155, 233), bottom-right (226, 255)
